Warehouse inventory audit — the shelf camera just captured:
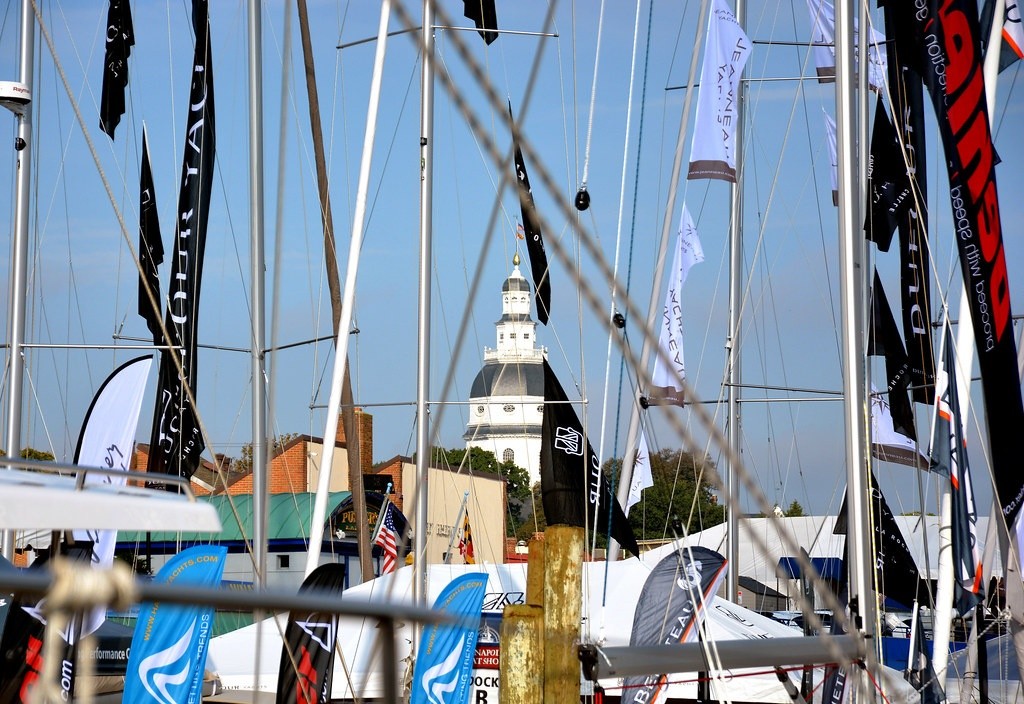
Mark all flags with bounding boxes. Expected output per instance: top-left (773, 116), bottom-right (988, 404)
top-left (688, 0), bottom-right (753, 182)
top-left (463, 0), bottom-right (498, 45)
top-left (831, 467), bottom-right (929, 610)
top-left (99, 0), bottom-right (135, 140)
top-left (807, 0), bottom-right (914, 253)
top-left (374, 504), bottom-right (396, 576)
top-left (459, 511), bottom-right (475, 565)
top-left (626, 432), bottom-right (653, 518)
top-left (138, 126), bottom-right (165, 352)
top-left (868, 268), bottom-right (917, 442)
top-left (509, 101), bottom-right (551, 325)
top-left (541, 358), bottom-right (639, 558)
top-left (652, 204), bottom-right (704, 391)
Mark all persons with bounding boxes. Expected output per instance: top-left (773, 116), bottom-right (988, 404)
top-left (988, 576), bottom-right (1006, 616)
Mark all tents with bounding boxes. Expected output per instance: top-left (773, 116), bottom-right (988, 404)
top-left (626, 516), bottom-right (1005, 611)
top-left (206, 564), bottom-right (921, 704)
